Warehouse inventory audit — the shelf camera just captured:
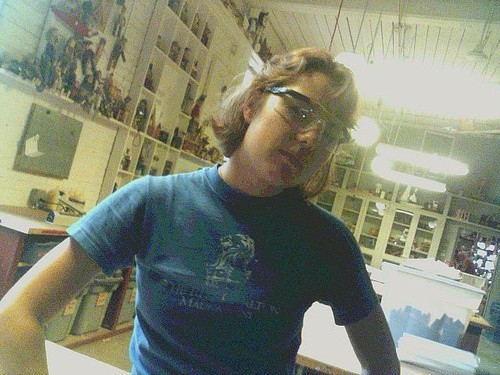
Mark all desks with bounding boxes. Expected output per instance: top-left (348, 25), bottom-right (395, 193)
top-left (371, 279), bottom-right (495, 353)
top-left (1, 203), bottom-right (78, 299)
top-left (298, 302), bottom-right (481, 375)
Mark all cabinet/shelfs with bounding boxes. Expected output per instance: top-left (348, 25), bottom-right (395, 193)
top-left (306, 130), bottom-right (499, 270)
top-left (96, 2), bottom-right (216, 209)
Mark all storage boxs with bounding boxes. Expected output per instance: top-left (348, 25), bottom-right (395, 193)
top-left (71, 265), bottom-right (125, 334)
top-left (27, 238), bottom-right (63, 266)
top-left (118, 264), bottom-right (139, 325)
top-left (42, 281), bottom-right (91, 343)
top-left (378, 261), bottom-right (488, 347)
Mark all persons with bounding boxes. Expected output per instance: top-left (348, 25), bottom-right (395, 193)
top-left (0, 46), bottom-right (403, 375)
top-left (6, 2), bottom-right (223, 165)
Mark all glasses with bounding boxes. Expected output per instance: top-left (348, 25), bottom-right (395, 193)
top-left (265, 86), bottom-right (353, 155)
